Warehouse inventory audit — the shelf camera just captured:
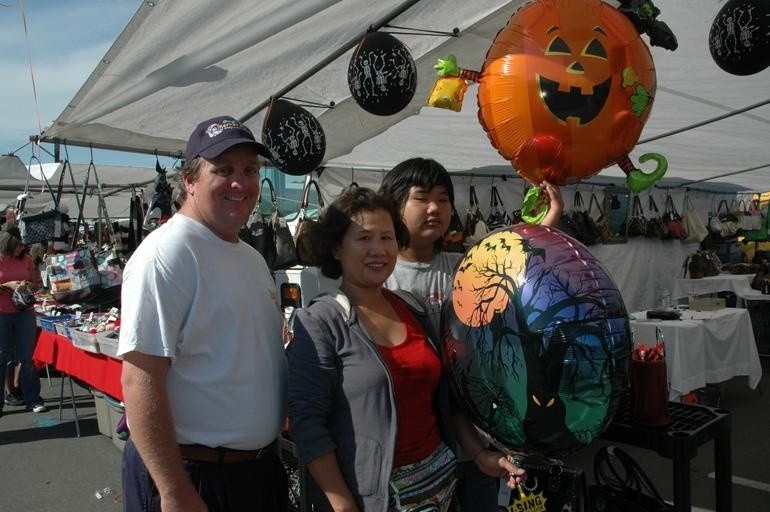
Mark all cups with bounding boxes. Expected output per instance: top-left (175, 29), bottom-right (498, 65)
top-left (658, 288), bottom-right (674, 308)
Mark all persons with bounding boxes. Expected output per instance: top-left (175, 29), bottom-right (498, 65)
top-left (4, 357), bottom-right (31, 409)
top-left (0, 227), bottom-right (49, 415)
top-left (289, 186), bottom-right (527, 511)
top-left (378, 158), bottom-right (567, 353)
top-left (122, 114), bottom-right (289, 510)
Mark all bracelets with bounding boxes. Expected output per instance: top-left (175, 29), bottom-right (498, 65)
top-left (471, 443), bottom-right (484, 459)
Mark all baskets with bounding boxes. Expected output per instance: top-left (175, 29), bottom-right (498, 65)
top-left (38, 313), bottom-right (78, 334)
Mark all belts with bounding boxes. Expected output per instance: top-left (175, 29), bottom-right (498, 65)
top-left (177, 445), bottom-right (272, 464)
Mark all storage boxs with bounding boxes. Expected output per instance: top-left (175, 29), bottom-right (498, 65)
top-left (56, 314), bottom-right (124, 360)
top-left (92, 389), bottom-right (125, 451)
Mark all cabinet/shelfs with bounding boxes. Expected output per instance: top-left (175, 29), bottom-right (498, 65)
top-left (603, 395), bottom-right (733, 511)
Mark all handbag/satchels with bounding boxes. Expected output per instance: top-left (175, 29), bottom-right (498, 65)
top-left (94, 245), bottom-right (130, 289)
top-left (440, 187), bottom-right (531, 253)
top-left (41, 250), bottom-right (100, 302)
top-left (556, 185), bottom-right (770, 296)
top-left (1, 281), bottom-right (36, 308)
top-left (383, 439), bottom-right (459, 512)
top-left (297, 180), bottom-right (332, 266)
top-left (498, 453), bottom-right (588, 512)
top-left (250, 177), bottom-right (296, 272)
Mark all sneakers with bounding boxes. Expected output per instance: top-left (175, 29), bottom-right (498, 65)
top-left (32, 403), bottom-right (46, 415)
top-left (5, 390), bottom-right (26, 407)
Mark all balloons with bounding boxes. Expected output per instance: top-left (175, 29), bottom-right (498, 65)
top-left (348, 26), bottom-right (419, 115)
top-left (709, 2), bottom-right (770, 77)
top-left (265, 98), bottom-right (323, 180)
top-left (430, 0), bottom-right (667, 218)
top-left (441, 218), bottom-right (636, 456)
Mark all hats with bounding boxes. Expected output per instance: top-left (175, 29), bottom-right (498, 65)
top-left (184, 115), bottom-right (274, 162)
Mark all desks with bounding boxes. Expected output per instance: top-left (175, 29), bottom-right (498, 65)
top-left (31, 330), bottom-right (125, 439)
top-left (671, 272), bottom-right (770, 307)
top-left (630, 308), bottom-right (763, 409)
